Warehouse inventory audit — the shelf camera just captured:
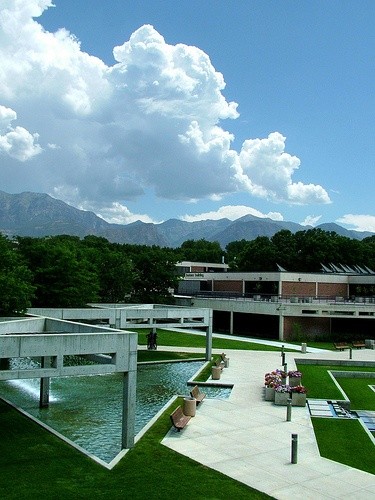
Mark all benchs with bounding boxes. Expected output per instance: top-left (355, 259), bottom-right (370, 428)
top-left (353, 341), bottom-right (366, 349)
top-left (170, 406), bottom-right (191, 432)
top-left (221, 353), bottom-right (229, 368)
top-left (215, 358), bottom-right (224, 370)
top-left (335, 342), bottom-right (349, 351)
top-left (190, 385), bottom-right (206, 406)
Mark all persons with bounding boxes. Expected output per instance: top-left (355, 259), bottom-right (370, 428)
top-left (145, 332), bottom-right (157, 350)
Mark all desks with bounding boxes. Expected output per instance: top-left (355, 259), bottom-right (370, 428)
top-left (183, 398), bottom-right (196, 417)
top-left (212, 365), bottom-right (221, 379)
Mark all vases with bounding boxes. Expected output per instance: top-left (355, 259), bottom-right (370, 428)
top-left (291, 392), bottom-right (306, 407)
top-left (274, 391), bottom-right (289, 405)
top-left (289, 376), bottom-right (301, 387)
top-left (265, 385), bottom-right (275, 401)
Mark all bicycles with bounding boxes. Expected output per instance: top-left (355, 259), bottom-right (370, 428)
top-left (146, 333), bottom-right (158, 351)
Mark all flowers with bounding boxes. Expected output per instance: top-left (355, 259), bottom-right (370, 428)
top-left (264, 369), bottom-right (307, 394)
